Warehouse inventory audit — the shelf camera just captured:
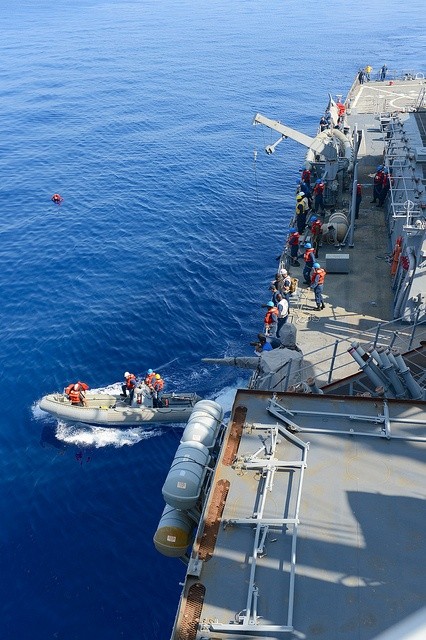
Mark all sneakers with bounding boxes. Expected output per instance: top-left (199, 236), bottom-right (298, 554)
top-left (303, 282), bottom-right (309, 284)
top-left (376, 204), bottom-right (382, 207)
top-left (120, 394), bottom-right (127, 397)
top-left (370, 200), bottom-right (376, 203)
top-left (321, 305), bottom-right (325, 308)
top-left (314, 308), bottom-right (321, 311)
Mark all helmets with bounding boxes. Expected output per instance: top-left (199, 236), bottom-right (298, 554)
top-left (147, 369), bottom-right (153, 374)
top-left (310, 216), bottom-right (317, 222)
top-left (304, 243), bottom-right (311, 248)
top-left (299, 192), bottom-right (305, 196)
top-left (377, 166), bottom-right (382, 171)
top-left (289, 228), bottom-right (297, 232)
top-left (267, 301), bottom-right (274, 306)
top-left (316, 179), bottom-right (321, 184)
top-left (296, 196), bottom-right (302, 200)
top-left (313, 263), bottom-right (320, 268)
top-left (155, 374), bottom-right (160, 379)
top-left (280, 269), bottom-right (287, 274)
top-left (124, 372), bottom-right (131, 377)
top-left (296, 179), bottom-right (300, 184)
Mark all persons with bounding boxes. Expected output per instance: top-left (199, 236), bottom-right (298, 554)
top-left (274, 274), bottom-right (283, 289)
top-left (358, 64), bottom-right (373, 84)
top-left (319, 109), bottom-right (342, 131)
top-left (313, 179), bottom-right (325, 212)
top-left (269, 285), bottom-right (279, 301)
top-left (294, 244), bottom-right (316, 286)
top-left (380, 64), bottom-right (388, 80)
top-left (145, 369), bottom-right (156, 389)
top-left (276, 294), bottom-right (289, 337)
top-left (120, 371), bottom-right (137, 402)
top-left (350, 182), bottom-right (361, 220)
top-left (264, 302), bottom-right (278, 324)
top-left (152, 373), bottom-right (164, 408)
top-left (256, 334), bottom-right (272, 352)
top-left (281, 268), bottom-right (291, 294)
top-left (294, 166), bottom-right (313, 234)
top-left (287, 227), bottom-right (299, 264)
top-left (306, 263), bottom-right (325, 311)
top-left (66, 383), bottom-right (86, 407)
top-left (310, 216), bottom-right (323, 258)
top-left (370, 165), bottom-right (393, 207)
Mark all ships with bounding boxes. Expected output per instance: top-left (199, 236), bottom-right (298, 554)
top-left (152, 68), bottom-right (426, 639)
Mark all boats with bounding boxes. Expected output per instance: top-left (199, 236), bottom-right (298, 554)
top-left (39, 390), bottom-right (203, 426)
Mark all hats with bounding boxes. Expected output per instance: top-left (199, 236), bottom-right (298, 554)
top-left (269, 285), bottom-right (275, 290)
top-left (74, 384), bottom-right (80, 391)
